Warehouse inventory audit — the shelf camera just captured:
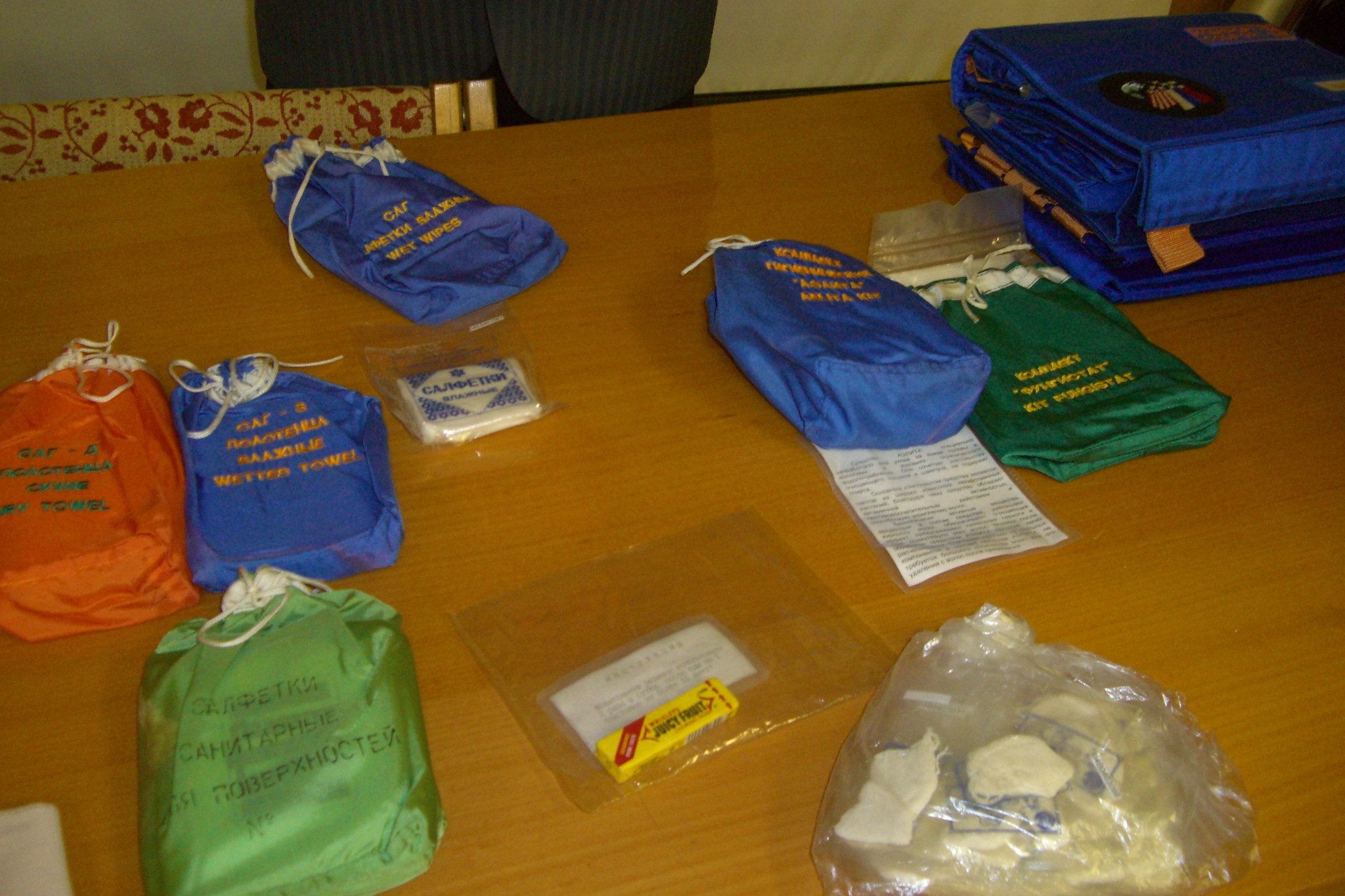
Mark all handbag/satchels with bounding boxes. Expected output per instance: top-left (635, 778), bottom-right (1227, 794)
top-left (908, 244), bottom-right (1231, 482)
top-left (0, 320), bottom-right (201, 640)
top-left (810, 603), bottom-right (1257, 896)
top-left (136, 565), bottom-right (445, 896)
top-left (680, 234), bottom-right (992, 449)
top-left (263, 134), bottom-right (568, 325)
top-left (170, 353), bottom-right (404, 593)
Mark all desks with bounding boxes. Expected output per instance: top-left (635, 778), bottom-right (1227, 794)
top-left (0, 83), bottom-right (1345, 896)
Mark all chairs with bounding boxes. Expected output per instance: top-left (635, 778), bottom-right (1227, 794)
top-left (0, 78), bottom-right (498, 181)
